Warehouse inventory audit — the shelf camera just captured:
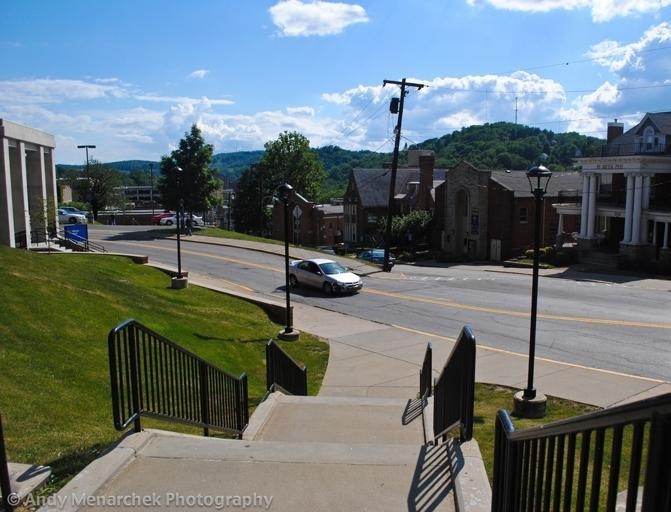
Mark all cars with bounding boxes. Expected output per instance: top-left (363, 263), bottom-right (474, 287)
top-left (360, 249), bottom-right (396, 266)
top-left (153, 211), bottom-right (177, 225)
top-left (57, 206), bottom-right (92, 224)
top-left (161, 213), bottom-right (204, 226)
top-left (288, 257), bottom-right (364, 296)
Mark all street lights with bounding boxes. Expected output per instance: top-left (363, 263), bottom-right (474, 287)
top-left (76, 145), bottom-right (97, 203)
top-left (171, 165), bottom-right (189, 289)
top-left (513, 158), bottom-right (556, 418)
top-left (278, 181), bottom-right (301, 342)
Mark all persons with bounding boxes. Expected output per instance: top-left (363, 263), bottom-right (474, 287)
top-left (186, 215), bottom-right (192, 236)
